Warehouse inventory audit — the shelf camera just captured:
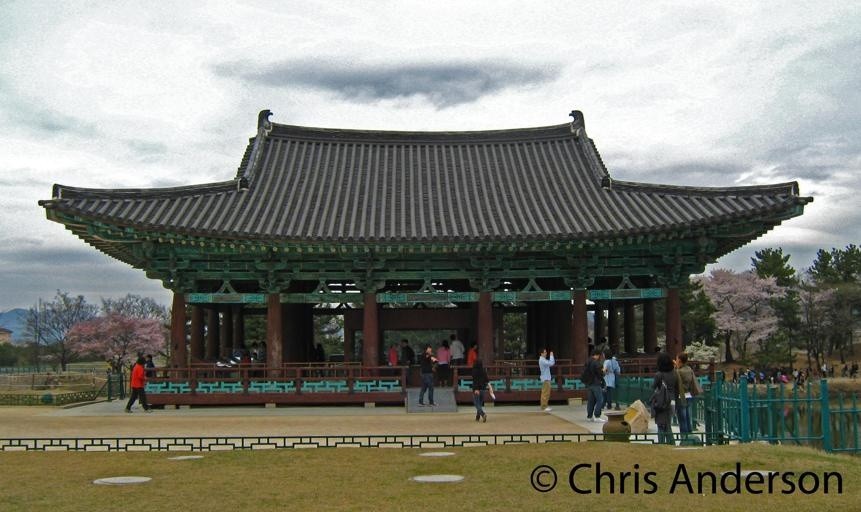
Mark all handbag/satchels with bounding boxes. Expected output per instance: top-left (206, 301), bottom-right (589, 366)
top-left (689, 369), bottom-right (703, 396)
top-left (648, 371), bottom-right (671, 410)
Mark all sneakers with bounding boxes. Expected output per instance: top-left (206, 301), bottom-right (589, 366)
top-left (543, 408), bottom-right (550, 411)
top-left (594, 418), bottom-right (605, 422)
top-left (482, 414), bottom-right (486, 423)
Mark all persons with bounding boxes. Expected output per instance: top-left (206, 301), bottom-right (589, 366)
top-left (119, 329), bottom-right (861, 447)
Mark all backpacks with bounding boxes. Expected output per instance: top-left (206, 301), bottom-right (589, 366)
top-left (580, 358), bottom-right (596, 385)
